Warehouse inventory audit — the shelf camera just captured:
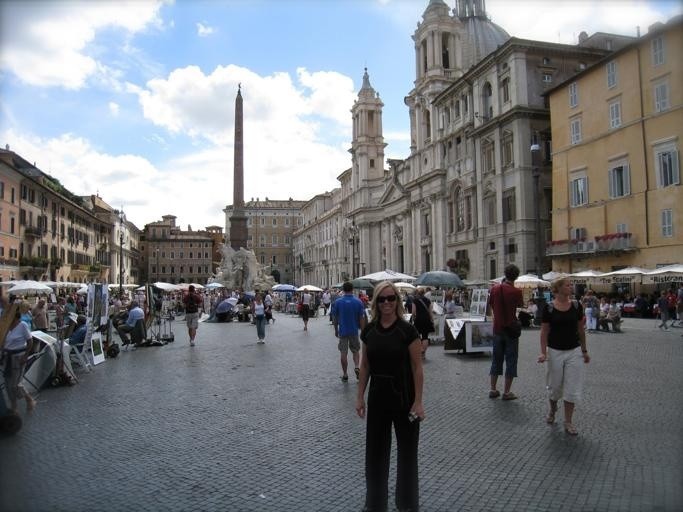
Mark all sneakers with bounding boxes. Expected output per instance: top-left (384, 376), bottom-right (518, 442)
top-left (355, 368), bottom-right (360, 379)
top-left (503, 392), bottom-right (518, 400)
top-left (342, 375), bottom-right (348, 382)
top-left (190, 339), bottom-right (195, 346)
top-left (257, 338), bottom-right (265, 343)
top-left (489, 391), bottom-right (500, 397)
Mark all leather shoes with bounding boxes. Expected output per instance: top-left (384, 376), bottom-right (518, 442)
top-left (565, 421), bottom-right (578, 435)
top-left (122, 341), bottom-right (130, 346)
top-left (547, 405), bottom-right (558, 423)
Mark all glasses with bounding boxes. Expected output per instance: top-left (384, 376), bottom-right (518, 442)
top-left (377, 294), bottom-right (396, 303)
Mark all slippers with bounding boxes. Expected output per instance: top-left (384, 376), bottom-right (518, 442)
top-left (26, 400), bottom-right (37, 416)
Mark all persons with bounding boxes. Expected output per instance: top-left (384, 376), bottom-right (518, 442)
top-left (485, 265), bottom-right (524, 401)
top-left (581, 282), bottom-right (683, 333)
top-left (405, 287), bottom-right (435, 341)
top-left (182, 285), bottom-right (203, 346)
top-left (202, 288), bottom-right (275, 344)
top-left (285, 288), bottom-right (330, 330)
top-left (1, 311), bottom-right (36, 420)
top-left (352, 281), bottom-right (427, 512)
top-left (21, 285), bottom-right (165, 346)
top-left (535, 272), bottom-right (591, 436)
top-left (444, 295), bottom-right (454, 314)
top-left (330, 282), bottom-right (366, 382)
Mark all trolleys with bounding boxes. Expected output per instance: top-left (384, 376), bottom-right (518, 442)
top-left (27, 325), bottom-right (67, 387)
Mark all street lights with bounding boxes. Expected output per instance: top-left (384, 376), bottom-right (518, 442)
top-left (530, 144), bottom-right (548, 325)
top-left (118, 212), bottom-right (125, 294)
top-left (349, 217), bottom-right (359, 280)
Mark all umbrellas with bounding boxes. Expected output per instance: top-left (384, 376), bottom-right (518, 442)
top-left (647, 265), bottom-right (673, 276)
top-left (542, 270), bottom-right (562, 280)
top-left (601, 267), bottom-right (647, 278)
top-left (659, 263), bottom-right (683, 277)
top-left (411, 270), bottom-right (467, 289)
top-left (354, 268), bottom-right (418, 282)
top-left (513, 274), bottom-right (551, 288)
top-left (566, 270), bottom-right (605, 278)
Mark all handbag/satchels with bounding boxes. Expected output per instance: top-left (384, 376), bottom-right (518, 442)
top-left (106, 344), bottom-right (120, 357)
top-left (297, 304), bottom-right (302, 312)
top-left (507, 321), bottom-right (522, 338)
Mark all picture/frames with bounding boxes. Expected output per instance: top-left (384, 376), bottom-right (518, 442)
top-left (90, 333), bottom-right (106, 366)
top-left (465, 321), bottom-right (494, 354)
